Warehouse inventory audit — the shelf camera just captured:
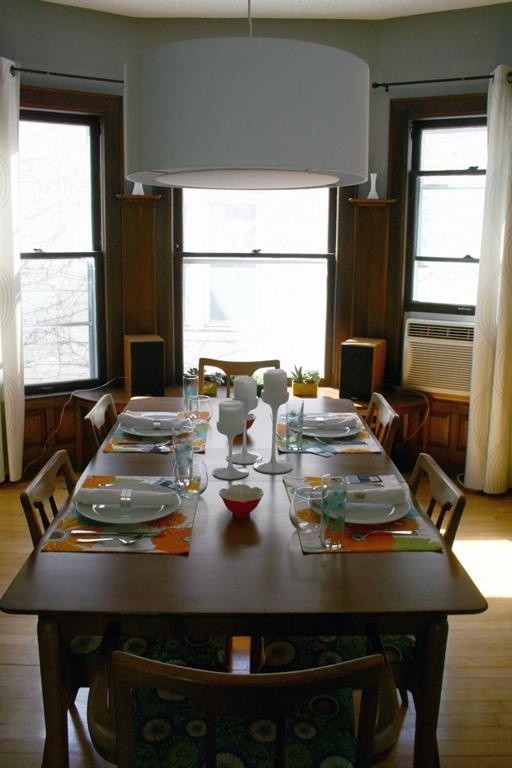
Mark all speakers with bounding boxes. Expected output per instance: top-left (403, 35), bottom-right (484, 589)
top-left (122, 333), bottom-right (166, 398)
top-left (339, 336), bottom-right (388, 404)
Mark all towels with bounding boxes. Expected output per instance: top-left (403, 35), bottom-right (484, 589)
top-left (303, 417), bottom-right (356, 428)
top-left (117, 412), bottom-right (189, 429)
top-left (313, 487), bottom-right (405, 505)
top-left (76, 488), bottom-right (177, 507)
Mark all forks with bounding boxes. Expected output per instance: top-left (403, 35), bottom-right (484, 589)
top-left (75, 533), bottom-right (142, 546)
top-left (117, 439), bottom-right (170, 448)
top-left (315, 437), bottom-right (368, 449)
top-left (348, 528), bottom-right (414, 542)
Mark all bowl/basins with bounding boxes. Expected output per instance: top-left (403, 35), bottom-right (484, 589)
top-left (245, 413), bottom-right (258, 436)
top-left (218, 485), bottom-right (263, 517)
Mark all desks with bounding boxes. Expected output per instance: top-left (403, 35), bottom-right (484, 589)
top-left (260, 385), bottom-right (433, 450)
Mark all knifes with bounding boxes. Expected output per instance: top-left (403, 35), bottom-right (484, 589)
top-left (71, 526), bottom-right (166, 539)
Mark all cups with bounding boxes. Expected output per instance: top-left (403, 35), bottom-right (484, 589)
top-left (319, 477), bottom-right (349, 550)
top-left (285, 399), bottom-right (304, 452)
top-left (288, 487), bottom-right (327, 533)
top-left (187, 396), bottom-right (212, 424)
top-left (182, 375), bottom-right (201, 417)
top-left (172, 426), bottom-right (194, 477)
top-left (278, 415), bottom-right (297, 444)
top-left (173, 457), bottom-right (209, 498)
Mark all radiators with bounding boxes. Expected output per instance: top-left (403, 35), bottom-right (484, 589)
top-left (401, 313), bottom-right (474, 397)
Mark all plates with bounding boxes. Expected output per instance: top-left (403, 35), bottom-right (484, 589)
top-left (76, 482), bottom-right (183, 526)
top-left (291, 413), bottom-right (363, 438)
top-left (119, 415), bottom-right (191, 439)
top-left (307, 482), bottom-right (414, 526)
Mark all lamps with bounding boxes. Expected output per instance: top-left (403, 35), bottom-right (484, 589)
top-left (121, 1), bottom-right (371, 193)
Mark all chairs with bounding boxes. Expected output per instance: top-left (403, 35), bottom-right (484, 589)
top-left (107, 648), bottom-right (384, 768)
top-left (408, 452), bottom-right (466, 548)
top-left (197, 357), bottom-right (281, 397)
top-left (364, 391), bottom-right (399, 457)
top-left (84, 393), bottom-right (118, 451)
top-left (20, 450), bottom-right (77, 549)
